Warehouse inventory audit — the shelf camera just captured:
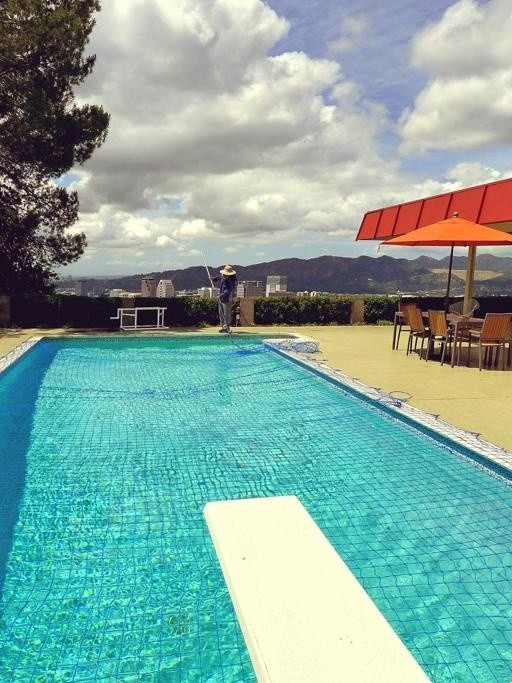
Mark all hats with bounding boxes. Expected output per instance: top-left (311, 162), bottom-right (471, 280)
top-left (220, 265), bottom-right (236, 275)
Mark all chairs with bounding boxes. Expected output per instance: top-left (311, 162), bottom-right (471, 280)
top-left (391, 300), bottom-right (512, 376)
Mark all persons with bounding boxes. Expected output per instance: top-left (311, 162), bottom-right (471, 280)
top-left (209, 266), bottom-right (236, 333)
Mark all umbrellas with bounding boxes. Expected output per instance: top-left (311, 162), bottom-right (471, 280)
top-left (378, 212), bottom-right (512, 358)
